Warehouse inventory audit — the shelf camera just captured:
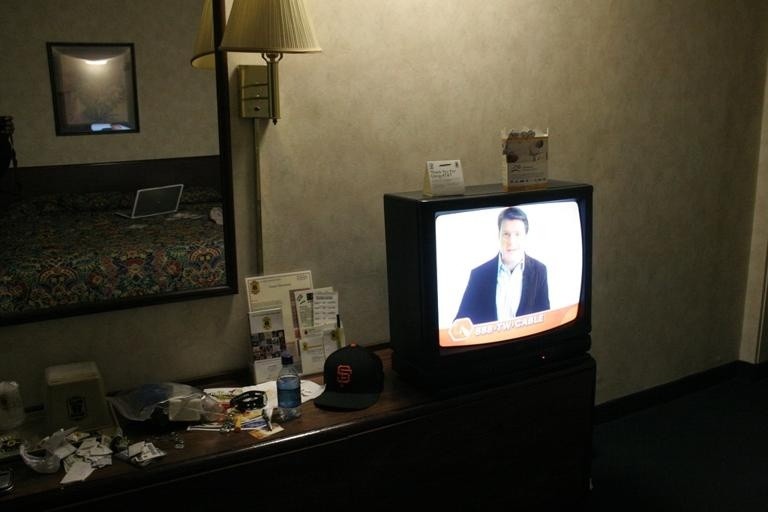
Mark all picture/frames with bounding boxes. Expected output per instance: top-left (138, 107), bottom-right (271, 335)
top-left (45, 39), bottom-right (143, 135)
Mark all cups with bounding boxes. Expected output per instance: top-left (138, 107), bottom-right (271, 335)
top-left (0, 381), bottom-right (28, 432)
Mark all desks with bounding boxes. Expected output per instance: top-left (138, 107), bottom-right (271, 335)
top-left (0, 346), bottom-right (598, 512)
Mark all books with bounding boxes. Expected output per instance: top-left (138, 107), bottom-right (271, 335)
top-left (247, 307), bottom-right (289, 364)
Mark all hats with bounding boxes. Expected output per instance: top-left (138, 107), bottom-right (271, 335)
top-left (313, 344), bottom-right (383, 411)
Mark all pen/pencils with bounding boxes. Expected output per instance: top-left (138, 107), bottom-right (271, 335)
top-left (262, 409), bottom-right (273, 431)
top-left (336, 315), bottom-right (342, 349)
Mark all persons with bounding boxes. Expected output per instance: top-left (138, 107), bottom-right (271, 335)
top-left (452, 206), bottom-right (551, 330)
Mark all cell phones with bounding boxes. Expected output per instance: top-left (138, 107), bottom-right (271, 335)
top-left (0, 466), bottom-right (17, 496)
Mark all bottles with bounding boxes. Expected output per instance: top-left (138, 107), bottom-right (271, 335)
top-left (276, 354), bottom-right (303, 422)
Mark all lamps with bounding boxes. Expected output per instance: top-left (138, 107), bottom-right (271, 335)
top-left (218, 1), bottom-right (324, 128)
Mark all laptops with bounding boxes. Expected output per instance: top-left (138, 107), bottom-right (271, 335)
top-left (114, 184), bottom-right (187, 220)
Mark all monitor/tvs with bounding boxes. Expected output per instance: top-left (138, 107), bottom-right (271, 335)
top-left (382, 178), bottom-right (594, 397)
